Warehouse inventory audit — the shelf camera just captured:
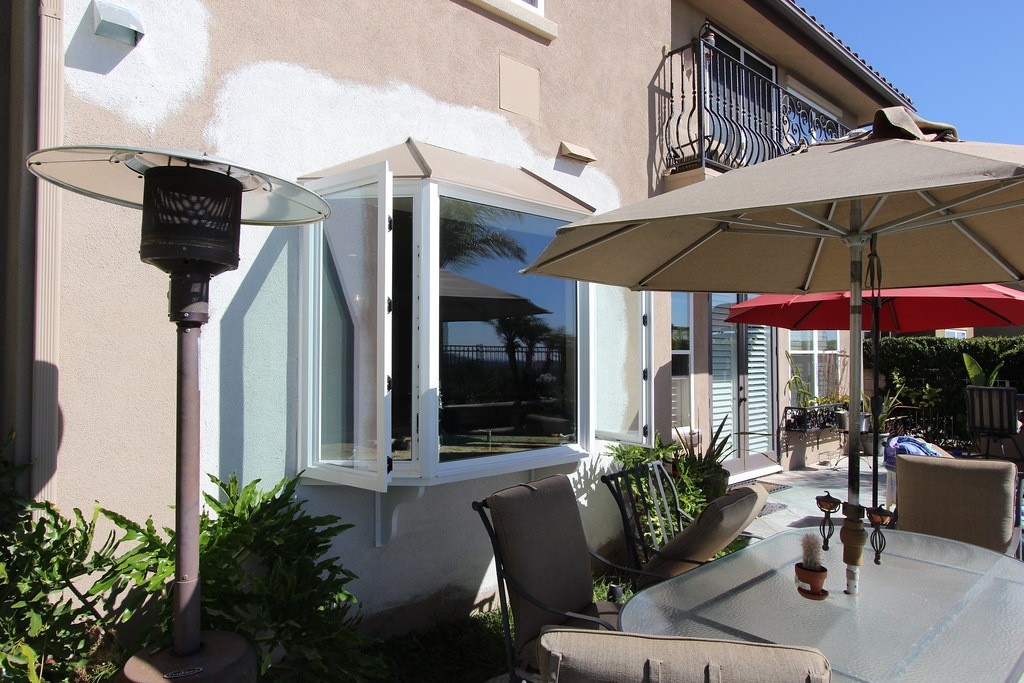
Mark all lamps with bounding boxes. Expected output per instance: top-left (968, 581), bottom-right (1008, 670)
top-left (23, 144), bottom-right (331, 682)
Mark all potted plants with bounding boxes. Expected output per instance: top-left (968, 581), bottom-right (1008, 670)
top-left (784, 351), bottom-right (851, 431)
top-left (794, 533), bottom-right (830, 601)
top-left (860, 383), bottom-right (905, 456)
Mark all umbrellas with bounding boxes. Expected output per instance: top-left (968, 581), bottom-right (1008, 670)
top-left (518, 106), bottom-right (1023, 519)
top-left (438, 266), bottom-right (554, 323)
top-left (724, 281), bottom-right (1023, 509)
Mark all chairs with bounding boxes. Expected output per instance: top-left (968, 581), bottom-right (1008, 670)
top-left (600, 461), bottom-right (767, 593)
top-left (967, 385), bottom-right (1024, 461)
top-left (536, 625), bottom-right (833, 683)
top-left (472, 473), bottom-right (674, 683)
top-left (892, 453), bottom-right (1023, 563)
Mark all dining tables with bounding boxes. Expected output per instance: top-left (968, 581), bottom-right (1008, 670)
top-left (616, 525), bottom-right (1023, 683)
